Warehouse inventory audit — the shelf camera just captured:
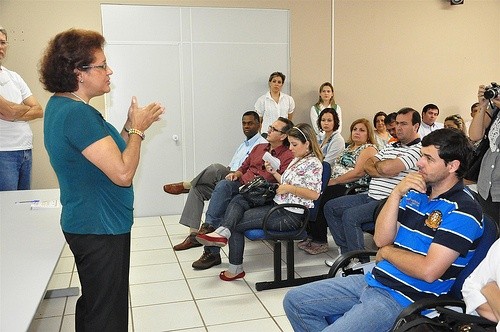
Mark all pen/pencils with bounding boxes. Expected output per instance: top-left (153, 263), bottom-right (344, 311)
top-left (15, 200), bottom-right (40, 203)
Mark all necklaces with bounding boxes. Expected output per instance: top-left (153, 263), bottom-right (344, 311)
top-left (71, 92), bottom-right (87, 104)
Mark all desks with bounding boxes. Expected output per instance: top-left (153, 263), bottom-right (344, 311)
top-left (0, 188), bottom-right (79, 332)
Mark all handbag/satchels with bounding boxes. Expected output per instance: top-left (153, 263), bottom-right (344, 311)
top-left (238, 175), bottom-right (279, 207)
top-left (463, 108), bottom-right (500, 182)
top-left (391, 306), bottom-right (497, 332)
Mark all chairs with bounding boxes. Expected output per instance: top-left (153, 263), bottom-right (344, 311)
top-left (326, 213), bottom-right (500, 332)
top-left (343, 184), bottom-right (389, 236)
top-left (243, 160), bottom-right (333, 293)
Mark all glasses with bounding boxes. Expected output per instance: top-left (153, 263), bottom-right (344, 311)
top-left (0, 41), bottom-right (8, 46)
top-left (268, 126), bottom-right (285, 134)
top-left (82, 63), bottom-right (108, 72)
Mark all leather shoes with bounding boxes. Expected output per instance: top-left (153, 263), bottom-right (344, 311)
top-left (164, 182), bottom-right (190, 195)
top-left (174, 236), bottom-right (203, 250)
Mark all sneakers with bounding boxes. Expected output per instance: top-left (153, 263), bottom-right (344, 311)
top-left (190, 223), bottom-right (215, 245)
top-left (191, 252), bottom-right (221, 270)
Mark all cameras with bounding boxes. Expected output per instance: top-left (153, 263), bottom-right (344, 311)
top-left (484, 82), bottom-right (500, 99)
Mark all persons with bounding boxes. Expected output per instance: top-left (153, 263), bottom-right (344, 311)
top-left (282, 126), bottom-right (485, 332)
top-left (0, 26), bottom-right (45, 191)
top-left (164, 111), bottom-right (269, 250)
top-left (195, 123), bottom-right (325, 282)
top-left (191, 117), bottom-right (294, 270)
top-left (255, 72), bottom-right (295, 138)
top-left (310, 81), bottom-right (342, 134)
top-left (462, 236), bottom-right (500, 332)
top-left (37, 29), bottom-right (165, 332)
top-left (298, 101), bottom-right (489, 281)
top-left (468, 85), bottom-right (500, 228)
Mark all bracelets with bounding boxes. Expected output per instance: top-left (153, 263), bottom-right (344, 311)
top-left (123, 125), bottom-right (129, 132)
top-left (128, 129), bottom-right (145, 140)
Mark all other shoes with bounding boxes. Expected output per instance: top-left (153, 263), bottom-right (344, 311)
top-left (195, 234), bottom-right (227, 248)
top-left (325, 254), bottom-right (342, 267)
top-left (220, 271), bottom-right (245, 281)
top-left (304, 243), bottom-right (329, 254)
top-left (298, 241), bottom-right (310, 249)
top-left (335, 258), bottom-right (361, 276)
